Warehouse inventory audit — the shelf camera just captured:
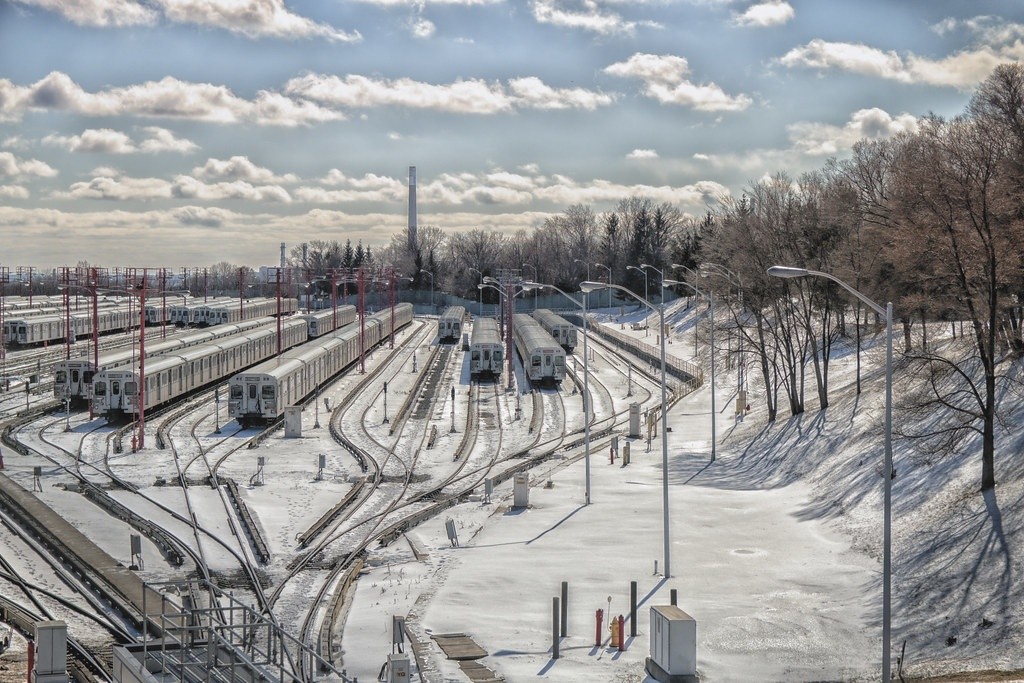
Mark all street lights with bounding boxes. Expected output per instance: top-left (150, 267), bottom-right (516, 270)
top-left (574, 259), bottom-right (590, 311)
top-left (626, 265), bottom-right (648, 337)
top-left (595, 264), bottom-right (611, 318)
top-left (95, 287), bottom-right (192, 449)
top-left (642, 264), bottom-right (664, 304)
top-left (523, 263), bottom-right (537, 309)
top-left (475, 276), bottom-right (533, 388)
top-left (699, 262), bottom-right (744, 422)
top-left (664, 279), bottom-right (715, 461)
top-left (672, 263), bottom-right (699, 357)
top-left (767, 265), bottom-right (894, 683)
top-left (580, 280), bottom-right (670, 578)
top-left (469, 268), bottom-right (483, 317)
top-left (522, 281), bottom-right (591, 505)
top-left (0, 268), bottom-right (433, 374)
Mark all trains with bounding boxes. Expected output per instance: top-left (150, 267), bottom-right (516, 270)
top-left (472, 317), bottom-right (503, 379)
top-left (437, 306), bottom-right (473, 345)
top-left (510, 312), bottom-right (566, 388)
top-left (532, 308), bottom-right (577, 354)
top-left (0, 294), bottom-right (415, 429)
